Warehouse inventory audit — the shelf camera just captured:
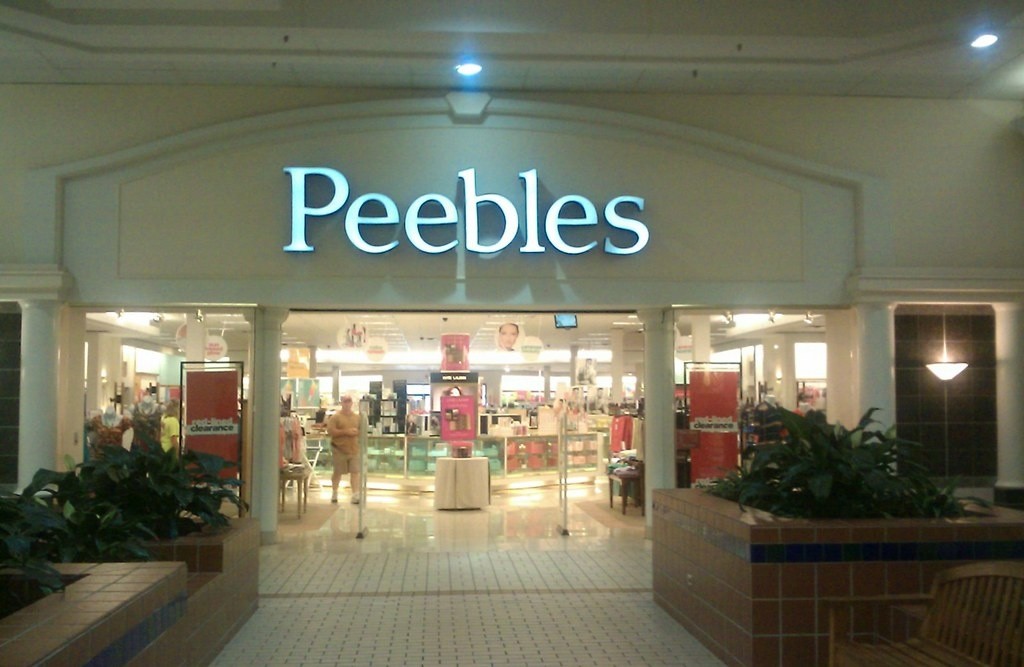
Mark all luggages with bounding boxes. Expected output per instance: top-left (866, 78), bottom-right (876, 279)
top-left (331, 495), bottom-right (337, 502)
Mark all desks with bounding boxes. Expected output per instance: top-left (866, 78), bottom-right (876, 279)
top-left (435, 457), bottom-right (490, 511)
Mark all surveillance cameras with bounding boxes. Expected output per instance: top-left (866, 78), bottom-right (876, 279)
top-left (442, 317), bottom-right (448, 321)
top-left (638, 329), bottom-right (642, 333)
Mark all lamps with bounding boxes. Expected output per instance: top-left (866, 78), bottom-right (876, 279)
top-left (722, 312), bottom-right (732, 323)
top-left (804, 312), bottom-right (812, 324)
top-left (769, 308), bottom-right (776, 324)
top-left (279, 343), bottom-right (290, 365)
top-left (195, 309), bottom-right (203, 323)
top-left (925, 313), bottom-right (968, 381)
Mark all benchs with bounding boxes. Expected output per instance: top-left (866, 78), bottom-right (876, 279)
top-left (830, 560), bottom-right (1024, 667)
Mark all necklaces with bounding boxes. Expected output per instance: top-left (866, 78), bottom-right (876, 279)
top-left (103, 414), bottom-right (116, 429)
top-left (142, 403), bottom-right (155, 416)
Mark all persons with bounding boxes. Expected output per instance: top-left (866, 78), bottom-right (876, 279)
top-left (558, 357), bottom-right (607, 415)
top-left (159, 398), bottom-right (182, 455)
top-left (84, 404), bottom-right (133, 461)
top-left (497, 323), bottom-right (520, 352)
top-left (326, 395), bottom-right (359, 505)
top-left (127, 394), bottom-right (167, 453)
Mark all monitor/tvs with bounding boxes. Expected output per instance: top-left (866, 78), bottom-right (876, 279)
top-left (554, 314), bottom-right (578, 328)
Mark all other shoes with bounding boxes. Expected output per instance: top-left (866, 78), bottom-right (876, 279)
top-left (352, 497), bottom-right (360, 504)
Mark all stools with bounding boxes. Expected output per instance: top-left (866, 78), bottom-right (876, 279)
top-left (609, 474), bottom-right (644, 517)
top-left (278, 473), bottom-right (310, 518)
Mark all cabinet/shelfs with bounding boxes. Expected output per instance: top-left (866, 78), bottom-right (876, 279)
top-left (378, 399), bottom-right (409, 433)
